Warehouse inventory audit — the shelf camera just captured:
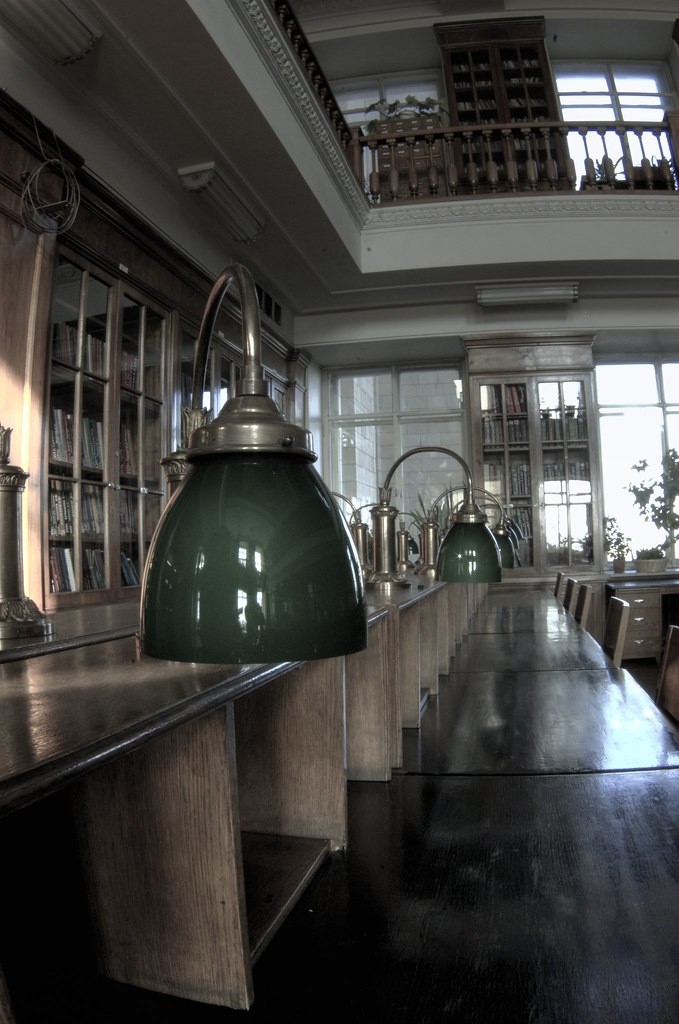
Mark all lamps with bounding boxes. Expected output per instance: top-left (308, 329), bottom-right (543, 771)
top-left (423, 487), bottom-right (524, 569)
top-left (178, 162), bottom-right (266, 246)
top-left (473, 281), bottom-right (580, 308)
top-left (374, 446), bottom-right (503, 584)
top-left (135, 263), bottom-right (369, 664)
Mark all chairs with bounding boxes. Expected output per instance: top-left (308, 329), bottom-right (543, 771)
top-left (654, 625), bottom-right (679, 724)
top-left (563, 578), bottom-right (578, 614)
top-left (602, 597), bottom-right (630, 668)
top-left (555, 571), bottom-right (565, 596)
top-left (574, 583), bottom-right (592, 628)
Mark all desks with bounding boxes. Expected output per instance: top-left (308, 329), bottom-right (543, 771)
top-left (450, 631), bottom-right (617, 672)
top-left (390, 669), bottom-right (679, 775)
top-left (467, 589), bottom-right (587, 634)
top-left (233, 768), bottom-right (678, 1024)
top-left (605, 581), bottom-right (679, 665)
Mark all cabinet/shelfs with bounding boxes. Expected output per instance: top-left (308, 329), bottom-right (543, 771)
top-left (0, 575), bottom-right (491, 1017)
top-left (467, 373), bottom-right (603, 577)
top-left (0, 87), bottom-right (312, 610)
top-left (433, 14), bottom-right (574, 195)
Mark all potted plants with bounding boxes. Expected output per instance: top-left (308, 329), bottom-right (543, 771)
top-left (608, 529), bottom-right (632, 571)
top-left (624, 447), bottom-right (679, 573)
top-left (366, 95), bottom-right (453, 203)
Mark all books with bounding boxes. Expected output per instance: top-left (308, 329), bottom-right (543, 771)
top-left (49, 546), bottom-right (140, 592)
top-left (53, 320), bottom-right (139, 391)
top-left (510, 509), bottom-right (532, 538)
top-left (492, 385), bottom-right (527, 413)
top-left (50, 408), bottom-right (137, 475)
top-left (49, 479), bottom-right (135, 536)
top-left (483, 415), bottom-right (588, 449)
top-left (454, 57), bottom-right (550, 151)
top-left (483, 462), bottom-right (590, 495)
